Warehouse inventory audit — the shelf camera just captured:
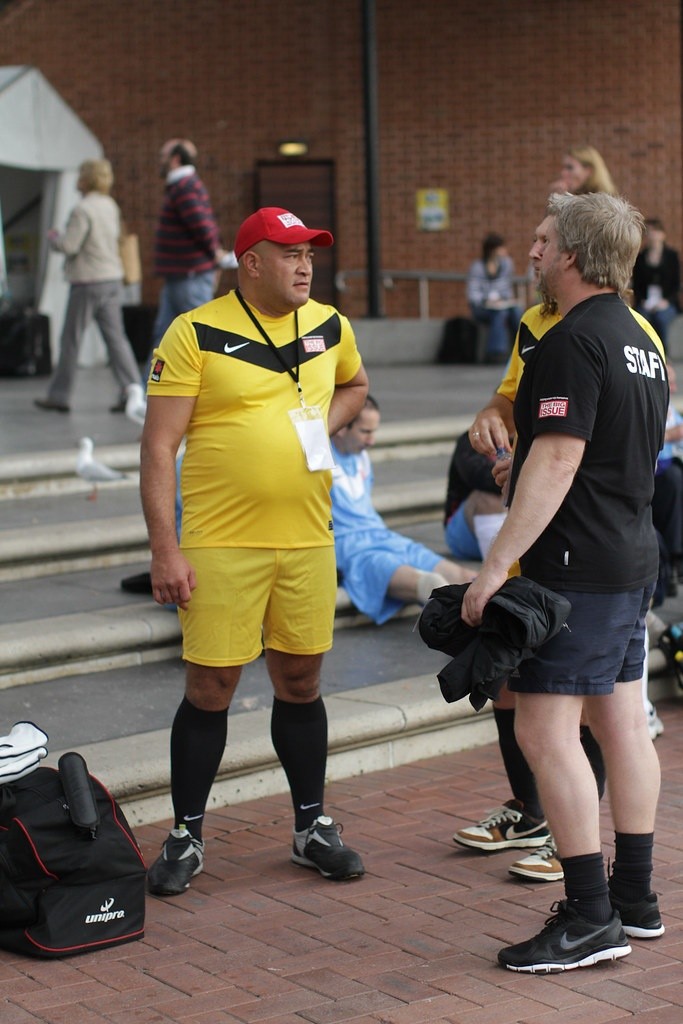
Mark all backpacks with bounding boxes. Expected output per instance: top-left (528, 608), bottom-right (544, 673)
top-left (438, 318), bottom-right (478, 364)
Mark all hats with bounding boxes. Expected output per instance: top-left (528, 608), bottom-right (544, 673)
top-left (233, 207), bottom-right (333, 261)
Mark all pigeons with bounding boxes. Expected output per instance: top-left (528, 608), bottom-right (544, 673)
top-left (72, 437), bottom-right (128, 501)
top-left (123, 383), bottom-right (147, 426)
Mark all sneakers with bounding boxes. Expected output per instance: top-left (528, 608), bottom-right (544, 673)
top-left (558, 859), bottom-right (665, 939)
top-left (290, 815), bottom-right (364, 880)
top-left (497, 900), bottom-right (632, 974)
top-left (452, 798), bottom-right (549, 851)
top-left (147, 823), bottom-right (206, 897)
top-left (508, 834), bottom-right (563, 881)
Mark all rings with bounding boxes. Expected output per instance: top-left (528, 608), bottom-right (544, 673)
top-left (473, 433), bottom-right (479, 436)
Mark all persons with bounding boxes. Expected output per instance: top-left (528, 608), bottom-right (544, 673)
top-left (460, 192), bottom-right (665, 973)
top-left (644, 608), bottom-right (683, 741)
top-left (464, 231), bottom-right (524, 363)
top-left (35, 158), bottom-right (142, 412)
top-left (451, 190), bottom-right (665, 881)
top-left (329, 396), bottom-right (480, 624)
top-left (560, 143), bottom-right (617, 199)
top-left (146, 139), bottom-right (230, 386)
top-left (633, 218), bottom-right (683, 354)
top-left (652, 364), bottom-right (683, 608)
top-left (141, 206), bottom-right (366, 897)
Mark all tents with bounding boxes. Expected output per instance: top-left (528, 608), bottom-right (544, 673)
top-left (0, 63), bottom-right (111, 364)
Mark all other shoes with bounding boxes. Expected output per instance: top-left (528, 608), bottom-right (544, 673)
top-left (109, 398), bottom-right (127, 413)
top-left (33, 396), bottom-right (72, 413)
top-left (649, 707), bottom-right (665, 741)
top-left (120, 573), bottom-right (154, 594)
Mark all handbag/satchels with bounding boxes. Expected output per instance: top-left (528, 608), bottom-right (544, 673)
top-left (0, 752), bottom-right (150, 958)
top-left (116, 208), bottom-right (143, 284)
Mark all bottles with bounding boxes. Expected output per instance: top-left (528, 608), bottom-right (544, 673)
top-left (496, 447), bottom-right (511, 463)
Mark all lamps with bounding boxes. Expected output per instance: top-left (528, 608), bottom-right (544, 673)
top-left (277, 124), bottom-right (315, 158)
